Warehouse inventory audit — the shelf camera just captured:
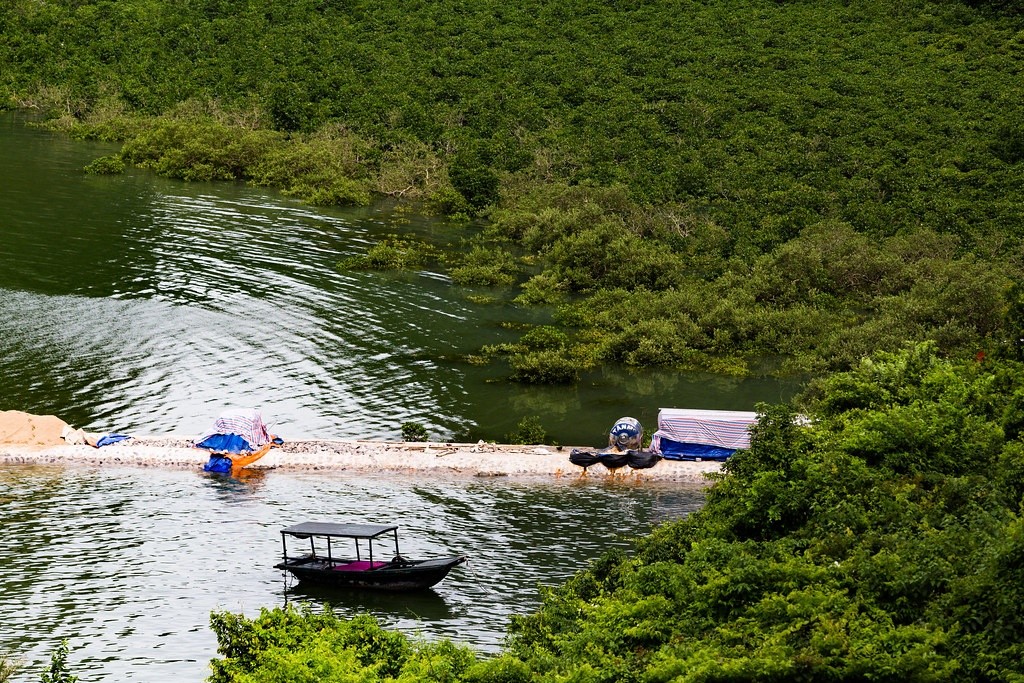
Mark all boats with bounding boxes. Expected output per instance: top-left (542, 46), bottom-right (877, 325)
top-left (271, 517), bottom-right (467, 592)
